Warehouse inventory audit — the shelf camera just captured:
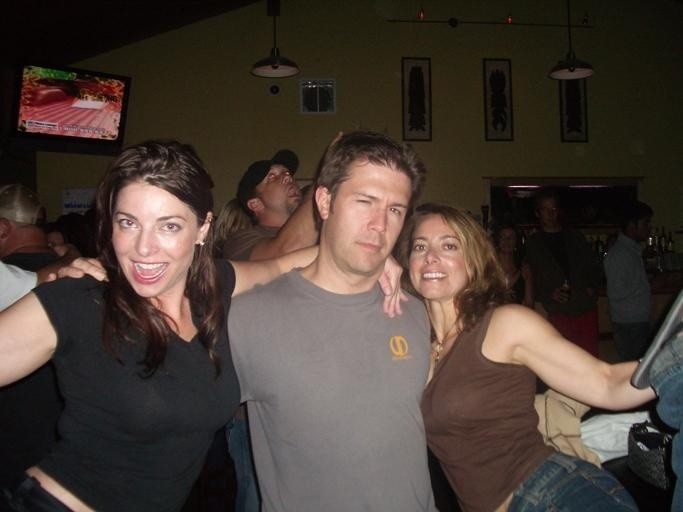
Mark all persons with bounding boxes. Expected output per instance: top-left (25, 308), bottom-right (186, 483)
top-left (1, 141), bottom-right (411, 512)
top-left (43, 131), bottom-right (438, 511)
top-left (0, 131), bottom-right (682, 364)
top-left (392, 200), bottom-right (683, 511)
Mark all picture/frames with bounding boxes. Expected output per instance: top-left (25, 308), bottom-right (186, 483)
top-left (558, 60), bottom-right (588, 143)
top-left (483, 58), bottom-right (514, 142)
top-left (401, 56), bottom-right (432, 142)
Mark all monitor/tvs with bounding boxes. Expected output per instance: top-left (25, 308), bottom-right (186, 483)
top-left (10, 61), bottom-right (133, 157)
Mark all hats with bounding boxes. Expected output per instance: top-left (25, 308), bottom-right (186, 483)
top-left (0, 184), bottom-right (49, 227)
top-left (236, 149), bottom-right (300, 206)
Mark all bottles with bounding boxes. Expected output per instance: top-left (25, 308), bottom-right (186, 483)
top-left (642, 227), bottom-right (675, 272)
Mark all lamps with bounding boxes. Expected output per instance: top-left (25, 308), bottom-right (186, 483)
top-left (548, 0), bottom-right (595, 80)
top-left (251, 0), bottom-right (300, 78)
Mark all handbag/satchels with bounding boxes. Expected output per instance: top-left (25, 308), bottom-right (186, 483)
top-left (627, 428), bottom-right (675, 495)
